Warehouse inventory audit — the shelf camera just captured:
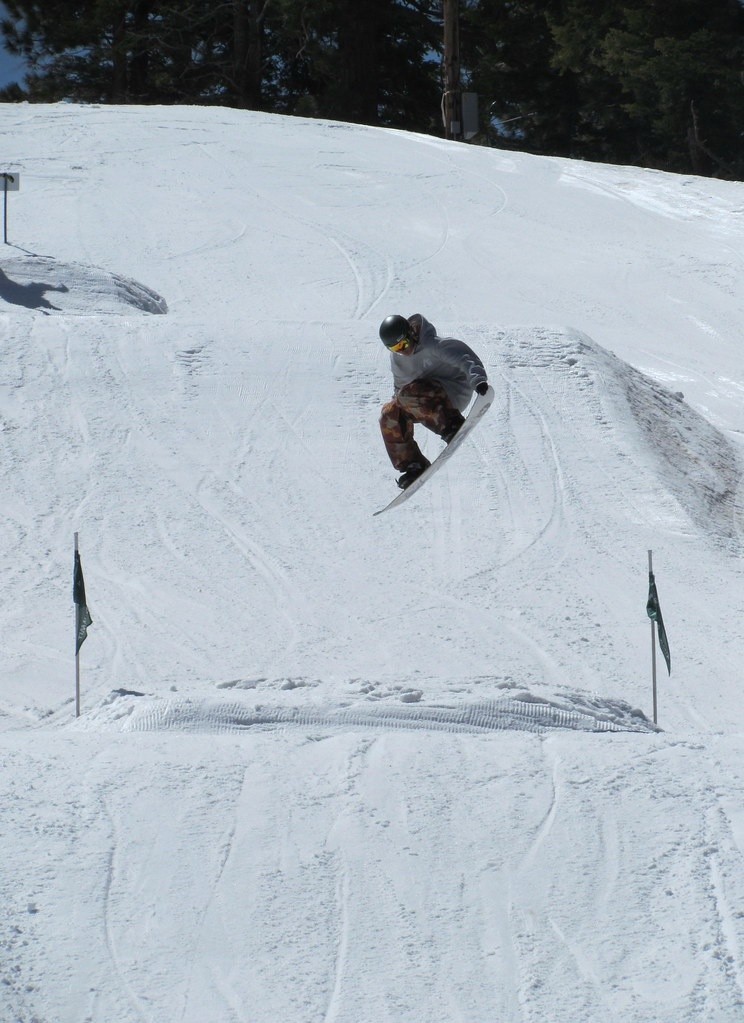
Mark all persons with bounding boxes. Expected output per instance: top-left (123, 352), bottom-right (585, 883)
top-left (378, 313), bottom-right (488, 488)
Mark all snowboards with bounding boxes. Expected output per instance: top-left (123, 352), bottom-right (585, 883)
top-left (372, 381), bottom-right (497, 516)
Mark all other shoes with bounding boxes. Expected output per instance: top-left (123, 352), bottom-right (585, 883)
top-left (395, 452), bottom-right (431, 489)
top-left (441, 408), bottom-right (466, 445)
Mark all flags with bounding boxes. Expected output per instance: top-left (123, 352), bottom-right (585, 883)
top-left (73, 551), bottom-right (93, 655)
top-left (646, 571), bottom-right (670, 677)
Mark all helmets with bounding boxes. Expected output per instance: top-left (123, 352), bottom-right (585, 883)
top-left (378, 315), bottom-right (409, 349)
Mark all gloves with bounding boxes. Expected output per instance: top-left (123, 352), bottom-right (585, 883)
top-left (476, 382), bottom-right (488, 396)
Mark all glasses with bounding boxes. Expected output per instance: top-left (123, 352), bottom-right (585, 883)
top-left (388, 337), bottom-right (411, 352)
